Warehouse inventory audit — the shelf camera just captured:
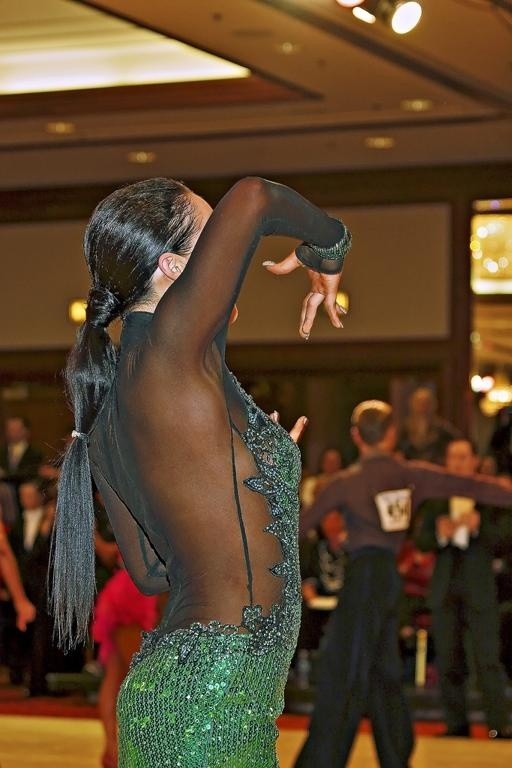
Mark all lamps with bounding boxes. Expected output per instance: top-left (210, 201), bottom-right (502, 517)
top-left (333, 0), bottom-right (424, 36)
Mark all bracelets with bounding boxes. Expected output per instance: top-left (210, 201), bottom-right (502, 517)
top-left (308, 215), bottom-right (353, 259)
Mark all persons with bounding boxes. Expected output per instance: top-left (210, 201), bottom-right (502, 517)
top-left (45, 174), bottom-right (354, 768)
top-left (295, 387), bottom-right (511, 768)
top-left (2, 416), bottom-right (160, 768)
